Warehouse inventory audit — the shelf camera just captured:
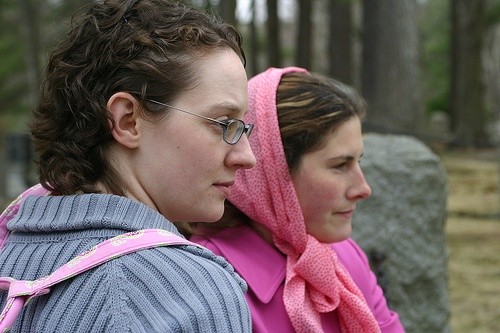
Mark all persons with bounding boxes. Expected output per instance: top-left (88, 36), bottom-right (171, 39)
top-left (188, 67), bottom-right (405, 333)
top-left (0, 0), bottom-right (257, 333)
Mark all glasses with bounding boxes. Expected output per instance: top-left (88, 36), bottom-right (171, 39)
top-left (127, 92), bottom-right (254, 145)
